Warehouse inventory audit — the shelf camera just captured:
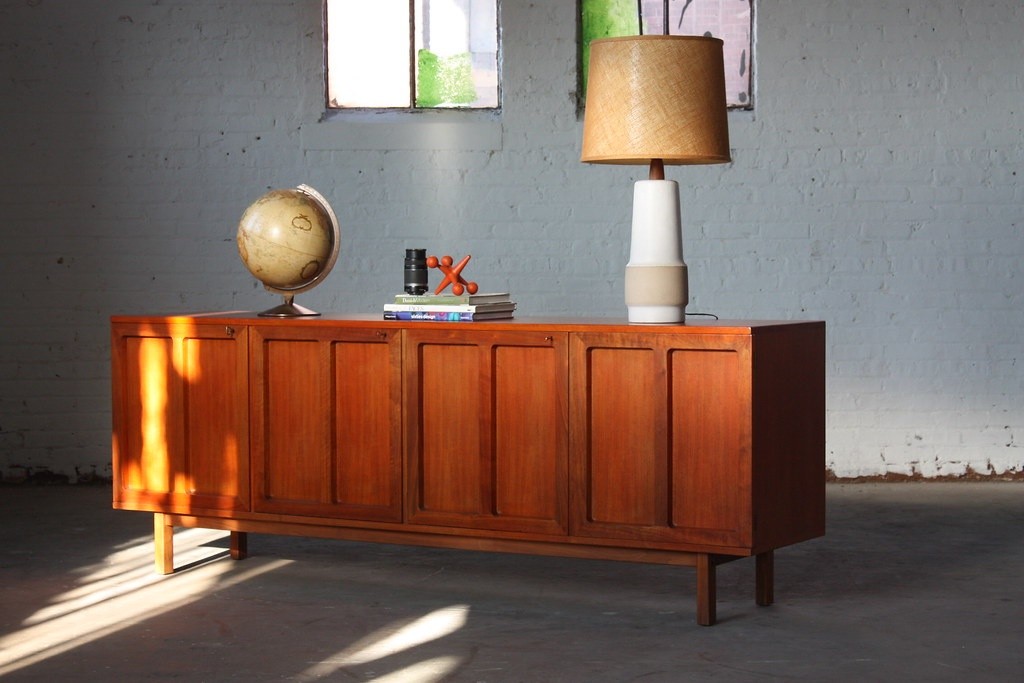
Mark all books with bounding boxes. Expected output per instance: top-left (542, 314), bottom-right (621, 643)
top-left (383, 312), bottom-right (516, 322)
top-left (383, 302), bottom-right (518, 312)
top-left (395, 292), bottom-right (511, 305)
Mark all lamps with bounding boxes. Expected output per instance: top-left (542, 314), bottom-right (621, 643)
top-left (579, 35), bottom-right (731, 324)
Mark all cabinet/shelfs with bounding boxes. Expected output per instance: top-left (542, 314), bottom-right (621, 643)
top-left (110, 310), bottom-right (828, 628)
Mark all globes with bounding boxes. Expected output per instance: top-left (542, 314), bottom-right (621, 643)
top-left (236, 182), bottom-right (341, 318)
